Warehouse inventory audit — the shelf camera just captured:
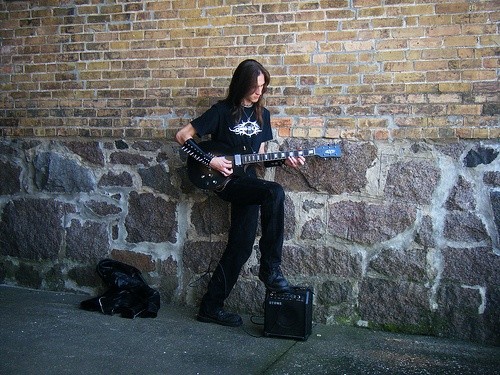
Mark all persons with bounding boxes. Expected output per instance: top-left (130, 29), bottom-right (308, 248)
top-left (176, 59), bottom-right (305, 326)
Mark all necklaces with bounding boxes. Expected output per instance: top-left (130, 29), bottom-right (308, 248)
top-left (241, 107), bottom-right (254, 122)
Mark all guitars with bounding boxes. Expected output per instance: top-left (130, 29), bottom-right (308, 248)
top-left (186, 139), bottom-right (343, 191)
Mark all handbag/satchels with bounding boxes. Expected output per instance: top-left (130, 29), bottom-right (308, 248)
top-left (79, 258), bottom-right (160, 319)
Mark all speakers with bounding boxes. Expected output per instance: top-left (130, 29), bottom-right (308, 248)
top-left (264, 286), bottom-right (313, 341)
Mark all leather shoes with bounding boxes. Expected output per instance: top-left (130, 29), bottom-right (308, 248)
top-left (197, 309), bottom-right (243, 327)
top-left (258, 266), bottom-right (289, 291)
